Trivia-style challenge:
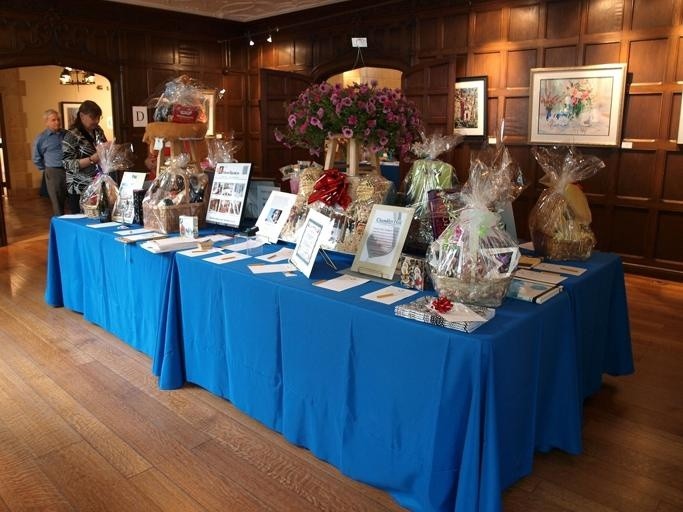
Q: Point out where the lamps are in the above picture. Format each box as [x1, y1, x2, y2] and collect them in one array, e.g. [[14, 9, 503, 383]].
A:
[[59, 66, 95, 93]]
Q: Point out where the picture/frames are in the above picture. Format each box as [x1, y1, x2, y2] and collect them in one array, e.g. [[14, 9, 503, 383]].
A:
[[62, 102, 83, 131], [453, 76, 488, 140], [195, 90, 217, 139], [527, 63, 627, 150]]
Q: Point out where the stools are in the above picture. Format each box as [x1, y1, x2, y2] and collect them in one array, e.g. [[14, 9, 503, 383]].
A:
[[146, 122, 208, 177], [323, 133, 381, 177]]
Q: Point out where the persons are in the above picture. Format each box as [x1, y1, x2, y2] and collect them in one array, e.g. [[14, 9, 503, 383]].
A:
[[270, 209, 281, 222], [400, 262, 421, 289], [32, 109, 78, 215], [61, 100, 108, 213]]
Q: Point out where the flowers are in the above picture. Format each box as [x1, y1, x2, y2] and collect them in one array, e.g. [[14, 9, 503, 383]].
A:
[[273, 79, 425, 161]]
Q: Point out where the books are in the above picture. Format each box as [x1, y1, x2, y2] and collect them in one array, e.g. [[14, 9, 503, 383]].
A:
[[140, 237, 198, 254], [394, 296, 495, 334], [114, 233, 162, 243], [505, 276, 563, 304], [517, 256, 540, 269]]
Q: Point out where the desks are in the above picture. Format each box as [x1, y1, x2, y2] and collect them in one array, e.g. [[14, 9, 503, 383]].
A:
[[44, 212, 635, 512]]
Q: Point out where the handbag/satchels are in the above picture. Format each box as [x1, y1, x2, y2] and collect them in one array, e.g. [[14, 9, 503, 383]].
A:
[[39, 172, 49, 198]]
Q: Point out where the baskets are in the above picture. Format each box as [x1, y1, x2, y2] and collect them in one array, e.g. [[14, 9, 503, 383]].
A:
[[404, 193, 495, 250], [431, 269, 512, 307], [79, 194, 114, 218], [530, 225, 594, 260], [141, 174, 209, 233]]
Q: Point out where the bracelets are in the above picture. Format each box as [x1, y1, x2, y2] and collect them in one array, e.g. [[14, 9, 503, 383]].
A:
[[89, 157, 95, 165]]
